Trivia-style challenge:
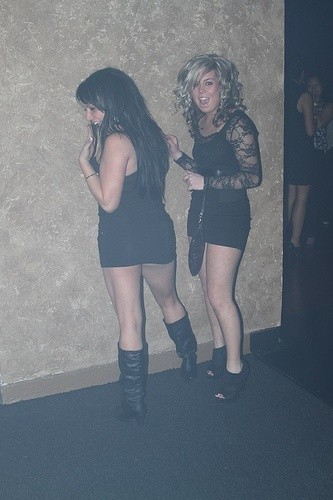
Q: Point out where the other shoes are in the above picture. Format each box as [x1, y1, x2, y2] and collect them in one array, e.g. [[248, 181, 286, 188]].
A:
[[284, 241, 316, 257]]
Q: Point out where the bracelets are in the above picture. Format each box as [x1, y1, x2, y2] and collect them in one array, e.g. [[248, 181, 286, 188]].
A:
[[85, 172, 98, 180]]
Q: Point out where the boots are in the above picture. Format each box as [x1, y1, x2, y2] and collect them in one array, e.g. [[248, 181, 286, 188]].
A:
[[163, 312, 196, 385], [106, 342, 148, 426]]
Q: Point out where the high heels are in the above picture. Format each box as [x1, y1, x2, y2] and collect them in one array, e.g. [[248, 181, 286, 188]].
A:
[[214, 360, 249, 401], [204, 344, 227, 378]]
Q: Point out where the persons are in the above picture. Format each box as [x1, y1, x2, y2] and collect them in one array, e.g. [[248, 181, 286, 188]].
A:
[[285, 55, 333, 260], [162, 55, 261, 404], [74, 68, 197, 422]]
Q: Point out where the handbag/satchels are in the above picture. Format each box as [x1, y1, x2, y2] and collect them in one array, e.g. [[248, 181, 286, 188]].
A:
[[187, 224, 205, 273]]
